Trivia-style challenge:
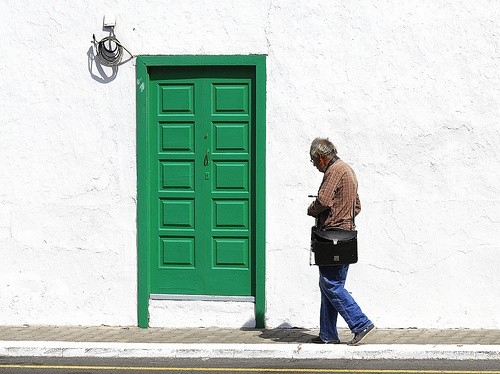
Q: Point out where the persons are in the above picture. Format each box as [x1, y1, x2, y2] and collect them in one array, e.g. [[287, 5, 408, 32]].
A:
[[308, 137, 375, 345]]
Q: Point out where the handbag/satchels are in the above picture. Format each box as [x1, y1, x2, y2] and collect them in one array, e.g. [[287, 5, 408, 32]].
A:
[[313, 230, 357, 266]]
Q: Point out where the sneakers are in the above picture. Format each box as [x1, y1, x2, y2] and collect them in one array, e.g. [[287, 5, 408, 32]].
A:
[[312, 336, 340, 344], [348, 324, 375, 346]]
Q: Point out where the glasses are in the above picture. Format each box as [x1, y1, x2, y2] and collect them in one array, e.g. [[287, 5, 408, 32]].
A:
[[310, 157, 319, 164]]
